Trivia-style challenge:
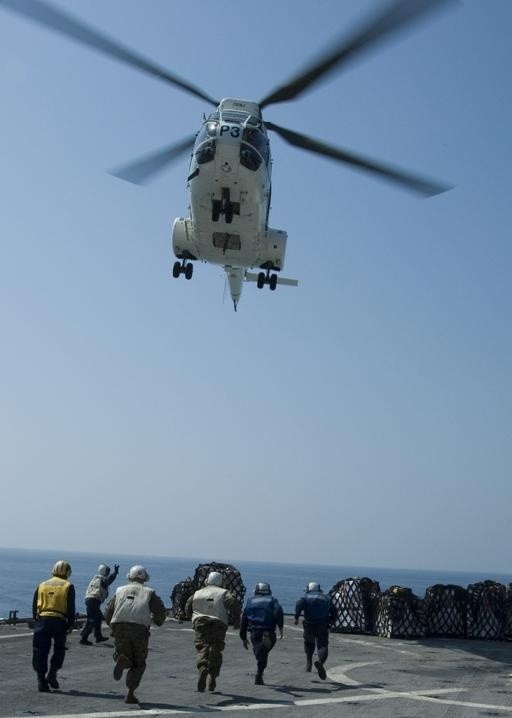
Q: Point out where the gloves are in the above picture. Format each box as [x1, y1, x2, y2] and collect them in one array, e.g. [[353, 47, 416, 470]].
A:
[[114, 563, 120, 573]]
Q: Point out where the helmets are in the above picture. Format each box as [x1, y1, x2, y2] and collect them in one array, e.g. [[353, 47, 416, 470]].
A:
[[205, 571, 225, 586], [51, 561, 72, 578], [98, 565, 110, 576], [307, 582, 321, 592], [255, 583, 272, 595], [127, 565, 149, 583]]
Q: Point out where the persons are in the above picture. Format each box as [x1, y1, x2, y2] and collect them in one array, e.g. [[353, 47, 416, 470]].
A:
[[239, 581, 286, 685], [295, 582, 339, 681], [31, 560, 77, 693], [79, 563, 121, 646], [102, 564, 166, 704], [184, 570, 241, 692]]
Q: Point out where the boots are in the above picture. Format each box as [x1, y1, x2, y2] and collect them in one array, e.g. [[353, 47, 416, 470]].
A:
[[197, 665, 208, 691], [209, 677, 215, 691], [124, 686, 140, 704], [314, 661, 326, 680], [79, 636, 93, 646], [95, 635, 109, 643], [305, 660, 312, 672], [113, 655, 126, 680], [47, 666, 60, 689], [37, 671, 50, 691], [254, 670, 264, 685]]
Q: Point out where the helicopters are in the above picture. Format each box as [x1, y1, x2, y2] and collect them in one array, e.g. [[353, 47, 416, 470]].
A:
[[0, 0, 467, 314]]
